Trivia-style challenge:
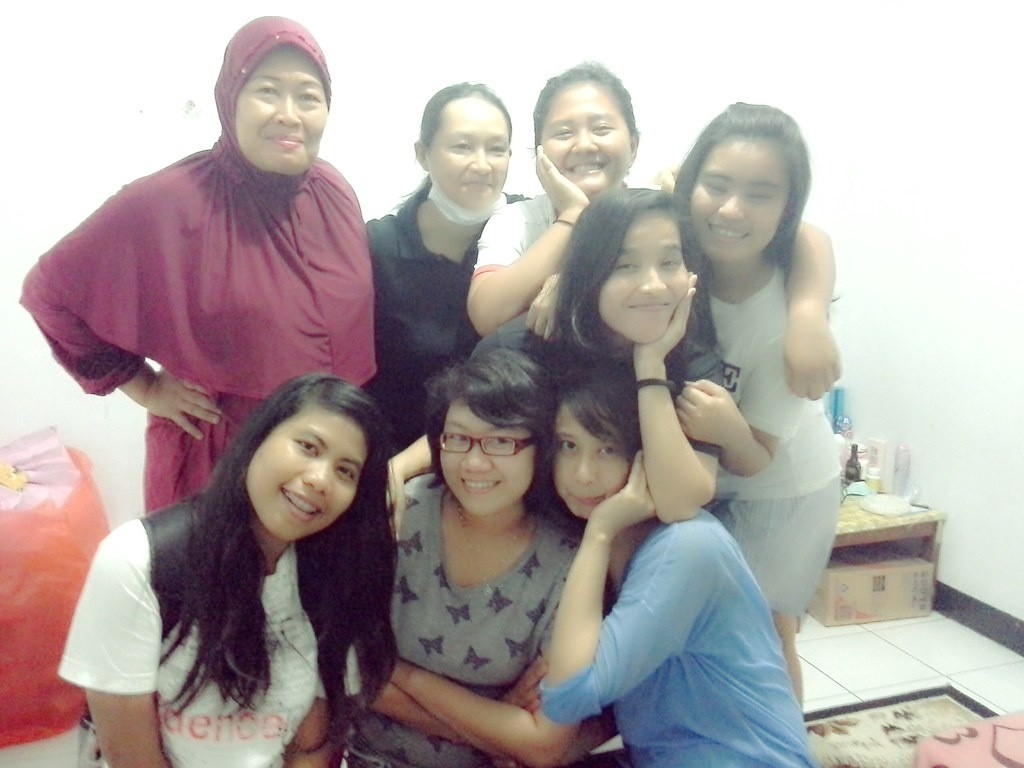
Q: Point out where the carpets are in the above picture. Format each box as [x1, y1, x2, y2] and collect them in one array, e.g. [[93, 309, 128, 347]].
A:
[[591, 686, 1000, 766]]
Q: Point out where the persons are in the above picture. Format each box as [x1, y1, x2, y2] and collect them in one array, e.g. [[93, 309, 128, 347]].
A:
[[382, 184, 723, 542], [523, 102, 846, 717], [535, 346, 815, 768], [345, 346, 579, 768], [306, 78, 686, 714], [59, 372, 403, 768], [17, 13, 380, 518], [466, 62, 846, 401]]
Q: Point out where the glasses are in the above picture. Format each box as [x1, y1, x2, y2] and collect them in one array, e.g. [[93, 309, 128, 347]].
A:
[[439, 433, 535, 456]]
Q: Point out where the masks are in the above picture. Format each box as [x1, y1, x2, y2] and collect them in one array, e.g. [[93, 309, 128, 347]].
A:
[[427, 151, 507, 225]]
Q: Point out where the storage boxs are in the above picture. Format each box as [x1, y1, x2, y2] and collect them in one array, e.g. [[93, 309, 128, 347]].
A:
[[805, 540, 936, 628]]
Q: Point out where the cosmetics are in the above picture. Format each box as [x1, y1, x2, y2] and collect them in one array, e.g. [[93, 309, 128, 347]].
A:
[[843, 437, 910, 499]]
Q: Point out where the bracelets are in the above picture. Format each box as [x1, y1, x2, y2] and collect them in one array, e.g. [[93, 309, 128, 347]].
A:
[[551, 220, 573, 227], [635, 378, 674, 391]]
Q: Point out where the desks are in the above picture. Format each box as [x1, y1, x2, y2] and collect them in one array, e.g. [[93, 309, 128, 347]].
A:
[[834, 499, 946, 564]]
